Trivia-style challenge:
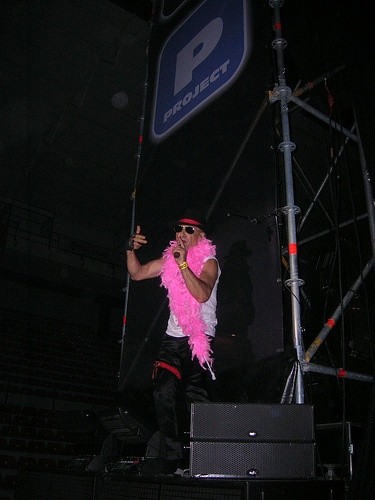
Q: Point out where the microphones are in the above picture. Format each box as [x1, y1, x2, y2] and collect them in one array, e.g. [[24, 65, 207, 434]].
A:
[[174, 239, 185, 257]]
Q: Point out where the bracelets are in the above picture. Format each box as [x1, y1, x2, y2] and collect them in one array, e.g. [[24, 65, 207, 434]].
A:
[[179, 261, 189, 271]]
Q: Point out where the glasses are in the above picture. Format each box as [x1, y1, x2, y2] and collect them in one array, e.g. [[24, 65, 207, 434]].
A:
[[173, 225, 198, 234]]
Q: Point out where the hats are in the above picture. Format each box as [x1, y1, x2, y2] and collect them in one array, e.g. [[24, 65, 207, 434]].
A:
[[170, 209, 210, 234]]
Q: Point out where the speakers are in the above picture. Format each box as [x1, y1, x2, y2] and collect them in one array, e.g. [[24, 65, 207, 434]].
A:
[[178, 404, 319, 477]]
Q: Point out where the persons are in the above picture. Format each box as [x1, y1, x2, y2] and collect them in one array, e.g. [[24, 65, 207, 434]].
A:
[[122, 214, 222, 474]]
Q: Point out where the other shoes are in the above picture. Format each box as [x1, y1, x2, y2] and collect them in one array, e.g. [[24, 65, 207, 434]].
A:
[[126, 458, 156, 473]]
